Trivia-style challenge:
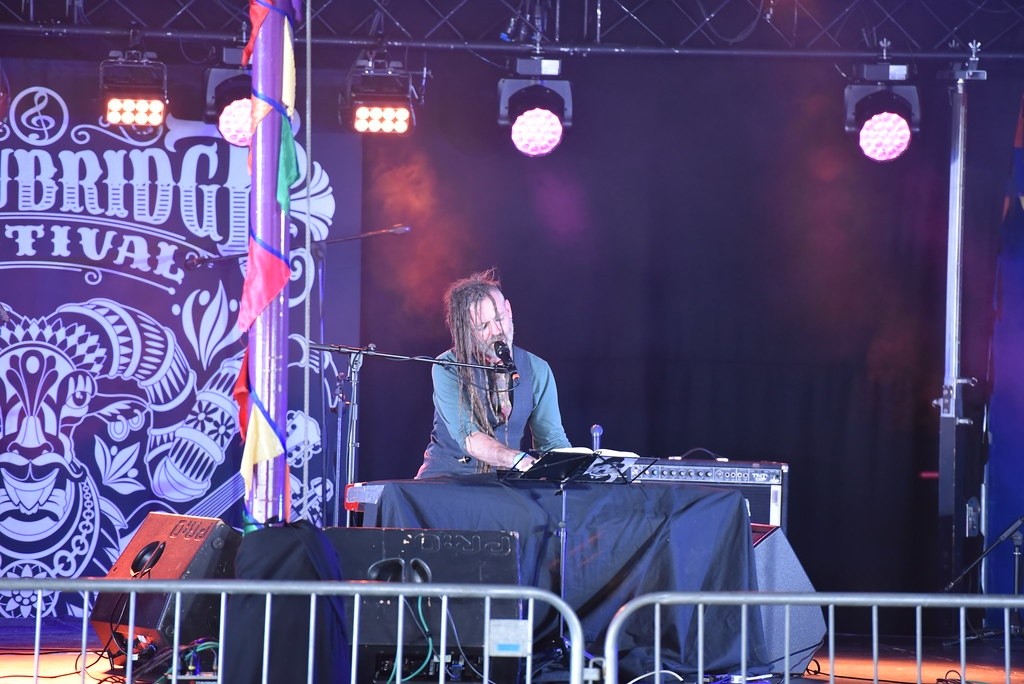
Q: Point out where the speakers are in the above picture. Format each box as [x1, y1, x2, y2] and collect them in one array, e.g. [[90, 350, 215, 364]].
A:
[[751, 524, 827, 675], [88, 510, 243, 677], [323, 526, 524, 684], [627, 456, 788, 532]]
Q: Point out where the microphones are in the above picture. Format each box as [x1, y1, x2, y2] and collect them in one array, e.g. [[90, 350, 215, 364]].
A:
[[184, 257, 204, 270], [494, 341, 521, 384]]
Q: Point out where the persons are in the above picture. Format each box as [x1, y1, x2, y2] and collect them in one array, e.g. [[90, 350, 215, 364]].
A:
[[413, 275, 572, 480]]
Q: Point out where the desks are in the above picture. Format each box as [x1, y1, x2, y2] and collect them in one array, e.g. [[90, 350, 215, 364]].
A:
[[374, 480, 776, 684]]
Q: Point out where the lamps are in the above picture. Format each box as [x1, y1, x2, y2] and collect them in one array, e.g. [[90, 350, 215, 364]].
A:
[[493, 54, 573, 159], [201, 44, 251, 147], [841, 57, 922, 162], [336, 48, 419, 137], [97, 47, 170, 127]]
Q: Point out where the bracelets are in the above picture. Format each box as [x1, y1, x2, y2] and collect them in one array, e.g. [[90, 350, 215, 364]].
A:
[[513, 453, 528, 468]]
